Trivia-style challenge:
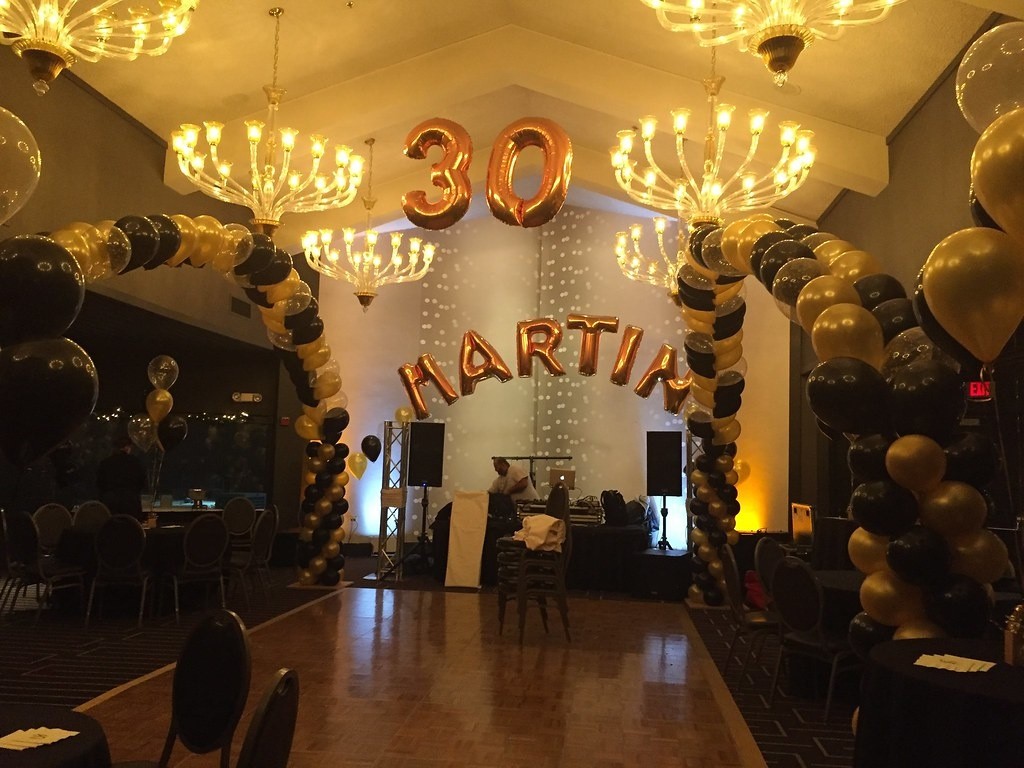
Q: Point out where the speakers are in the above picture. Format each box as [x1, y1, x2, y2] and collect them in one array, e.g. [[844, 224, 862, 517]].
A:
[[407, 422, 446, 488], [646, 431, 682, 497]]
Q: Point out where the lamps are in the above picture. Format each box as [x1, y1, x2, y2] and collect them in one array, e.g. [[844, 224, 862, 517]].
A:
[[0, 0, 196, 97], [640, 0, 905, 88], [614, 216, 691, 306], [170, 8, 365, 238], [301, 139, 436, 315], [609, 6, 818, 228]]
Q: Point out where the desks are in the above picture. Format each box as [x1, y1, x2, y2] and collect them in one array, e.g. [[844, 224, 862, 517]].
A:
[[633, 549, 690, 599], [812, 569, 865, 631], [0, 699, 111, 768], [140, 507, 265, 527]]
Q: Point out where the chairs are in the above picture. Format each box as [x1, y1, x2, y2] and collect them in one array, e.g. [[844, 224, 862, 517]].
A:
[[111, 608, 250, 768], [0, 496, 279, 625], [236, 667, 298, 768], [720, 537, 865, 727], [496, 482, 573, 644]]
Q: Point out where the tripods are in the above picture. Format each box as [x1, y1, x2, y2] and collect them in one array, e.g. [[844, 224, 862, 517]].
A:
[[654, 496, 674, 549], [380, 486, 432, 580]]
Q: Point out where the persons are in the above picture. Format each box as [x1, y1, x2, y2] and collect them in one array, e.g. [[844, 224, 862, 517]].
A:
[[97, 439, 148, 520], [488, 457, 539, 501]]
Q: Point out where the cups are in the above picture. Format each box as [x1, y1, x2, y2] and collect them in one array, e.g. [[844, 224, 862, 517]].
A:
[[160, 494, 172, 509], [149, 519, 156, 528]]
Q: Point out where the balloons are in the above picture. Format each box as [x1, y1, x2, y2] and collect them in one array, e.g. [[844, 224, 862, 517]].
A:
[[957, 21, 1024, 135], [402, 116, 572, 232], [362, 435, 381, 463], [348, 453, 367, 480], [677, 214, 1009, 738], [0, 104, 366, 586], [396, 407, 413, 428], [970, 184, 1005, 232], [922, 226, 1024, 365], [397, 314, 692, 421], [970, 107, 1023, 250]]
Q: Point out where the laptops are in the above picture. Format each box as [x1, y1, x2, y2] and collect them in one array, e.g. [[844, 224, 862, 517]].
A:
[[549, 468, 576, 490]]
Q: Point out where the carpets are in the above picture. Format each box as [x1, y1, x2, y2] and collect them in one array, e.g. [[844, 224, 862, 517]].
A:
[[342, 557, 444, 590], [686, 606, 857, 768], [0, 585, 350, 717]]
